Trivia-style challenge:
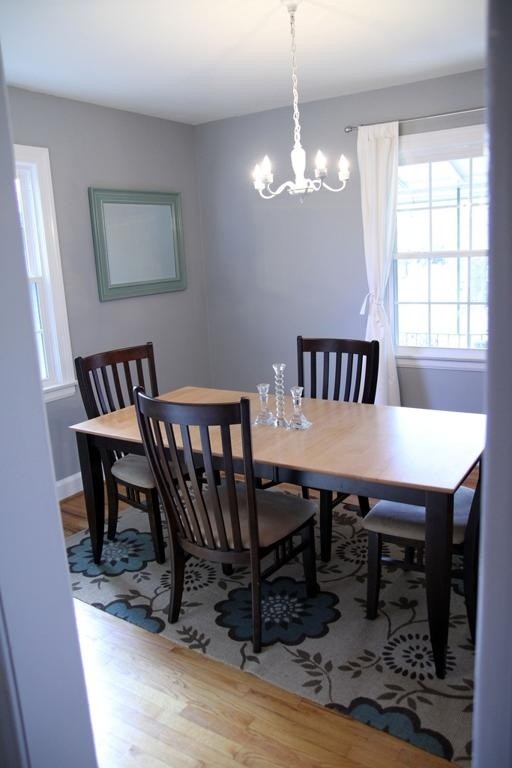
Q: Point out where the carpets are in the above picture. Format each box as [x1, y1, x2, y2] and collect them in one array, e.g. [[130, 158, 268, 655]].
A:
[[66, 472, 475, 766]]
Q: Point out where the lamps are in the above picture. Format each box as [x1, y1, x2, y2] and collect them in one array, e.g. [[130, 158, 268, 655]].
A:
[[251, 1, 350, 202]]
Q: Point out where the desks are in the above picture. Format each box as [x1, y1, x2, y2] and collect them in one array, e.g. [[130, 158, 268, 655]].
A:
[[68, 386, 487, 680]]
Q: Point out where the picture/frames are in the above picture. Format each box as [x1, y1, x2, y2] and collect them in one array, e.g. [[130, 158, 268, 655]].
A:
[[87, 187, 188, 303]]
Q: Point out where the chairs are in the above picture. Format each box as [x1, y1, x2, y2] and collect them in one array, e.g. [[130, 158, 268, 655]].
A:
[[130, 386, 320, 655], [297, 336, 379, 554], [75, 342, 221, 564], [363, 459, 481, 648]]
